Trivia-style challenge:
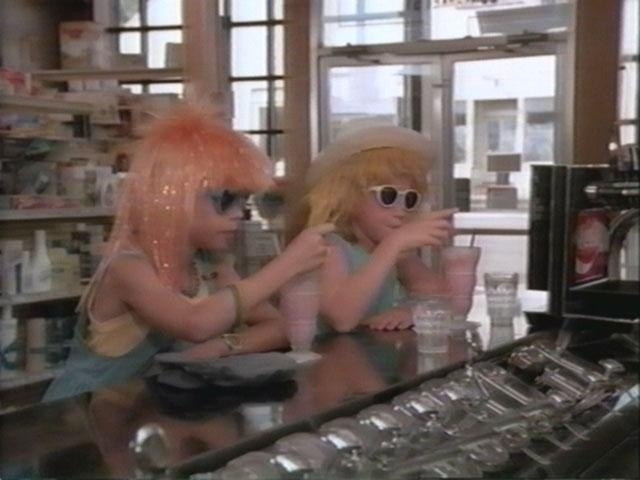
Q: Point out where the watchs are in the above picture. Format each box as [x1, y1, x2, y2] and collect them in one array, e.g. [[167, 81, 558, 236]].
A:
[[221, 333, 242, 353]]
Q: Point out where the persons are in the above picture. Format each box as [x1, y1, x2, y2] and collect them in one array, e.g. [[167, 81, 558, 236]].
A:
[[284, 324, 469, 425], [41, 112, 337, 405], [82, 383, 243, 479], [279, 124, 460, 335]]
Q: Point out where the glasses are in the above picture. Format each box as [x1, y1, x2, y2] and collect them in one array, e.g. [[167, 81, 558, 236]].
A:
[[361, 184, 422, 210], [205, 190, 235, 211]]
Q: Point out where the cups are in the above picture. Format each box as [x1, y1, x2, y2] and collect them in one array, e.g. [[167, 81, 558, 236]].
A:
[[280, 278, 322, 354], [409, 246, 520, 354]]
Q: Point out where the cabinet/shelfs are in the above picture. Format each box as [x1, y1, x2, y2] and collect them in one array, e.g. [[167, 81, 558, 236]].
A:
[[0, 88, 148, 418]]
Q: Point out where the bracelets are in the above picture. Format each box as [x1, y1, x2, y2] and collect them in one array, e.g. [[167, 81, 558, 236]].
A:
[[227, 280, 243, 331]]
